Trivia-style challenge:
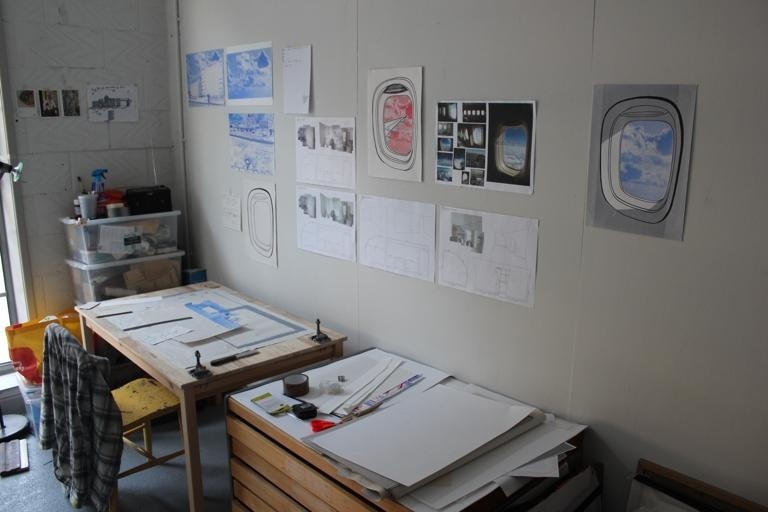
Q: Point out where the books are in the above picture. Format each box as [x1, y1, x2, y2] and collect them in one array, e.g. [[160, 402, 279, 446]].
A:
[[16, 371, 43, 440], [61, 211, 187, 309]]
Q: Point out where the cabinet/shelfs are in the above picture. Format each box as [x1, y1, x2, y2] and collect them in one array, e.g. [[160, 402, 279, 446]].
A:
[[73, 199, 81, 219], [106, 203, 124, 217], [87, 248, 97, 265]]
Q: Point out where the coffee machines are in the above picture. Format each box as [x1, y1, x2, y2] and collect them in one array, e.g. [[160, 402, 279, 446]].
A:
[[312, 420, 340, 432]]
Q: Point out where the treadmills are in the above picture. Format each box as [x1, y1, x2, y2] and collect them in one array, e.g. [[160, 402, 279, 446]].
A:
[[292, 402, 317, 421]]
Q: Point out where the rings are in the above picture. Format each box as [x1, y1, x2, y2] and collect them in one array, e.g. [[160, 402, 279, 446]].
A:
[[339, 400, 383, 424], [122, 316, 192, 332], [78, 176, 96, 194], [96, 311, 132, 318]]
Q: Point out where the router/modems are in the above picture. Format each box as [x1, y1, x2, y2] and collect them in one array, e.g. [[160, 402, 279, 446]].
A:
[[283, 373, 309, 398]]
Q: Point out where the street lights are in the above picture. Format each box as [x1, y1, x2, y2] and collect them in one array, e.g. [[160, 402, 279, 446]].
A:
[[5, 311, 83, 385]]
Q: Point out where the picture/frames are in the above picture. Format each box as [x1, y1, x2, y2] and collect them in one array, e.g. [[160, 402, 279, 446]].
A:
[[74, 281, 348, 512], [224, 347, 602, 511]]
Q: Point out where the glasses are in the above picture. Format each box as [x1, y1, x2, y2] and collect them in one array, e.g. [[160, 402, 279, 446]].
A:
[[1, 159, 29, 440]]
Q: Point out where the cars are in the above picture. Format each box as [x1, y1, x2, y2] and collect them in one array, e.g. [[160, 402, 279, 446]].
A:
[[77, 194, 100, 221]]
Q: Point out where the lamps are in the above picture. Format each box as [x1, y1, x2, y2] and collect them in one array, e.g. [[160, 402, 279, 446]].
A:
[[44, 324, 184, 512]]
